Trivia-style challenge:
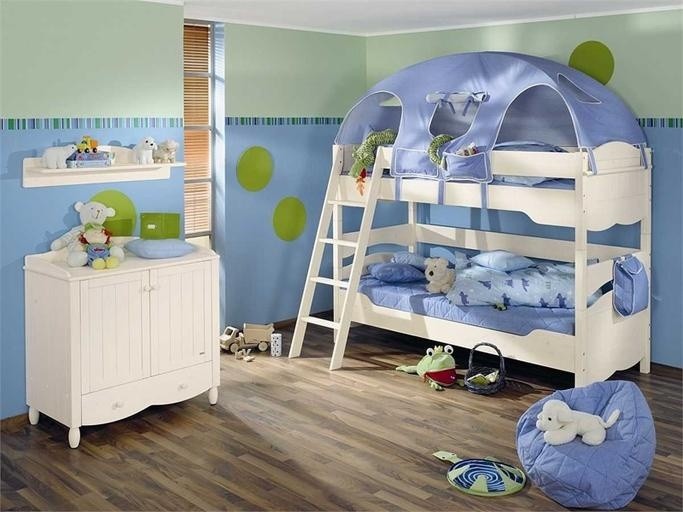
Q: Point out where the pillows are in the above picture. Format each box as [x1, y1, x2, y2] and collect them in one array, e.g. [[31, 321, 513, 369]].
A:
[[469, 248, 536, 273], [122, 238, 196, 260], [366, 250, 432, 284]]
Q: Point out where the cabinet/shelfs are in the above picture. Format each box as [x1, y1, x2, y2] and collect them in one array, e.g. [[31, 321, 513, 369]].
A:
[[20, 235, 221, 449]]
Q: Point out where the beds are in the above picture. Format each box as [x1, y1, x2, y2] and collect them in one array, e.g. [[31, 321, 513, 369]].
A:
[[326, 142, 653, 393]]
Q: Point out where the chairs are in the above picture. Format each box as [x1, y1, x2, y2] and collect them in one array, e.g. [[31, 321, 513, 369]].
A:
[[516, 379, 655, 510]]
